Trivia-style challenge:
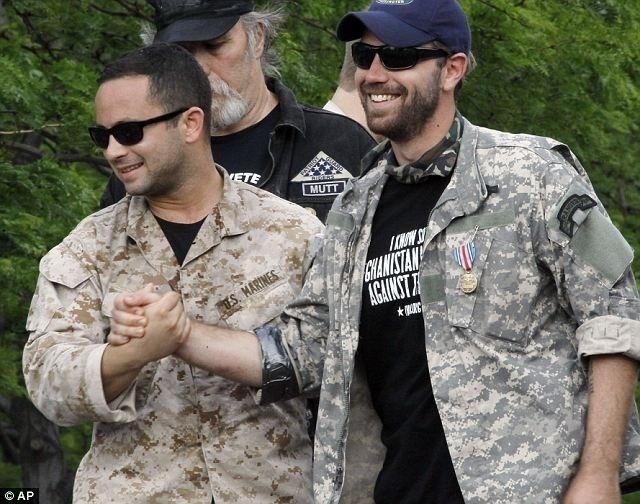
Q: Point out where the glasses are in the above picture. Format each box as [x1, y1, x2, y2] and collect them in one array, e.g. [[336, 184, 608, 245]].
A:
[[351, 42, 449, 72], [88, 107, 188, 150]]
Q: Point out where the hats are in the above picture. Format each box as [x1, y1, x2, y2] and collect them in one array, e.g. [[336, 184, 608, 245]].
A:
[[154, 0, 253, 43], [337, 1, 472, 55]]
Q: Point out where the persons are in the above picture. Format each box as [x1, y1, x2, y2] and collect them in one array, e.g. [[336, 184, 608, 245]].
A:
[[20, 41, 326, 504], [103, 0, 640, 503], [322, 3, 391, 146], [98, 0, 381, 224]]
